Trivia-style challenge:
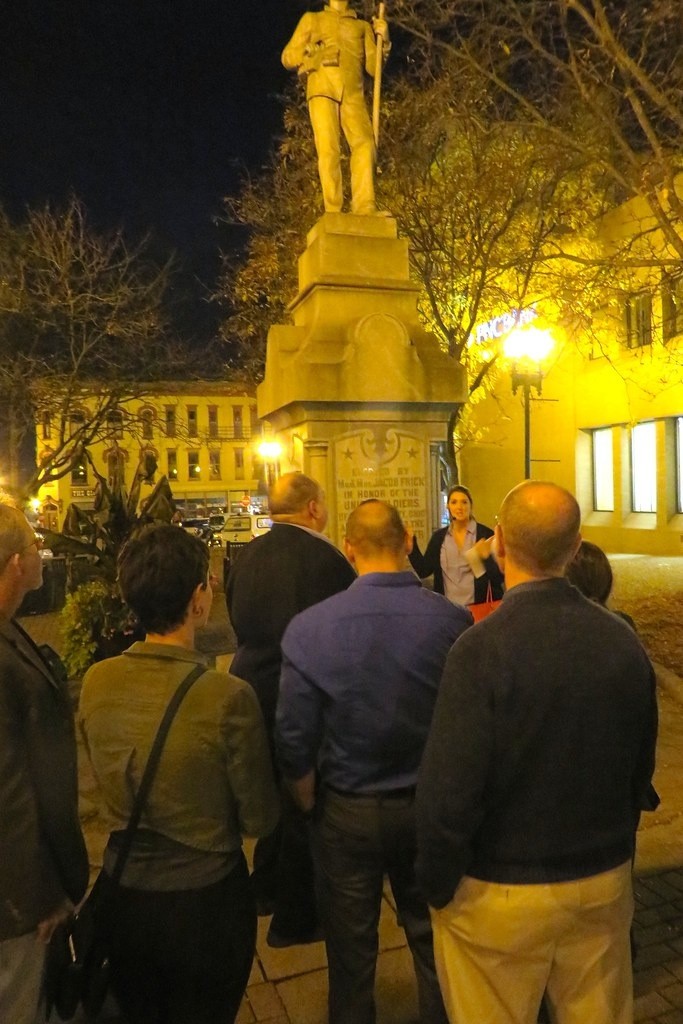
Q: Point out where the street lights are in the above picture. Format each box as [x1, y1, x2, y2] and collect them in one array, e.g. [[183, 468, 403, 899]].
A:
[[508, 329, 556, 477]]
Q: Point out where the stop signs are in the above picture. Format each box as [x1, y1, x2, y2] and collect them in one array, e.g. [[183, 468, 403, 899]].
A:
[[242, 496, 250, 506]]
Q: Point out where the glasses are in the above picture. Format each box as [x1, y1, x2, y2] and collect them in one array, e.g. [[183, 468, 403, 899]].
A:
[[4, 532, 45, 561]]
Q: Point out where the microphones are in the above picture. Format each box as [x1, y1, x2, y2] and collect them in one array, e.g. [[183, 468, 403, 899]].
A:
[[448, 508, 457, 521]]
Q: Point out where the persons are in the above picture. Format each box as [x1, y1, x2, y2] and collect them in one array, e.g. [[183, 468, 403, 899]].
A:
[[405, 484, 506, 608], [70, 516, 286, 1024], [269, 496, 477, 1024], [0, 492, 94, 1024], [280, 0, 394, 221], [223, 471, 360, 948], [406, 479, 657, 1024], [553, 538, 661, 964], [170, 509, 184, 527]]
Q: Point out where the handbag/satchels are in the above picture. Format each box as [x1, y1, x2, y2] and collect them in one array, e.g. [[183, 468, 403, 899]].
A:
[[46, 884, 113, 1018]]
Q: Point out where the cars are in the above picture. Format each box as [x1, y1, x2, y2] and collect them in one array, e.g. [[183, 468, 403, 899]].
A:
[[170, 512, 273, 547]]
